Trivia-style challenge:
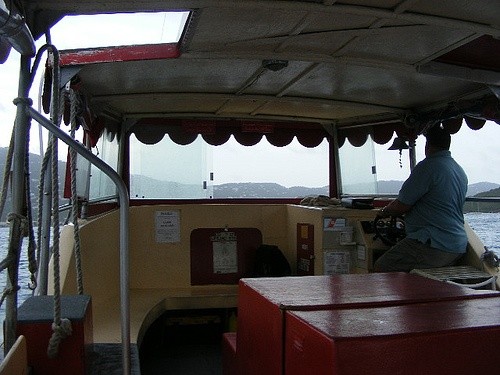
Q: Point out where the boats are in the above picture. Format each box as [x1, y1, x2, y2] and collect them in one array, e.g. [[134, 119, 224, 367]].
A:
[[0, 0, 499, 375]]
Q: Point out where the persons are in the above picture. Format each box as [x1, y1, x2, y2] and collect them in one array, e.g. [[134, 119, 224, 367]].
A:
[[369, 126, 468, 273]]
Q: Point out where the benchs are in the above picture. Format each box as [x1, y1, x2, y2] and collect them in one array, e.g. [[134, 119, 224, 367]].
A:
[[2, 293, 93, 374]]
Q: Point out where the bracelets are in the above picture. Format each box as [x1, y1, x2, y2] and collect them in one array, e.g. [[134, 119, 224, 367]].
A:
[[381, 206, 391, 217]]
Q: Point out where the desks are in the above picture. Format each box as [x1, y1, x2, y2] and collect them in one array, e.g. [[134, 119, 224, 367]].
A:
[[222, 264, 500, 375]]
